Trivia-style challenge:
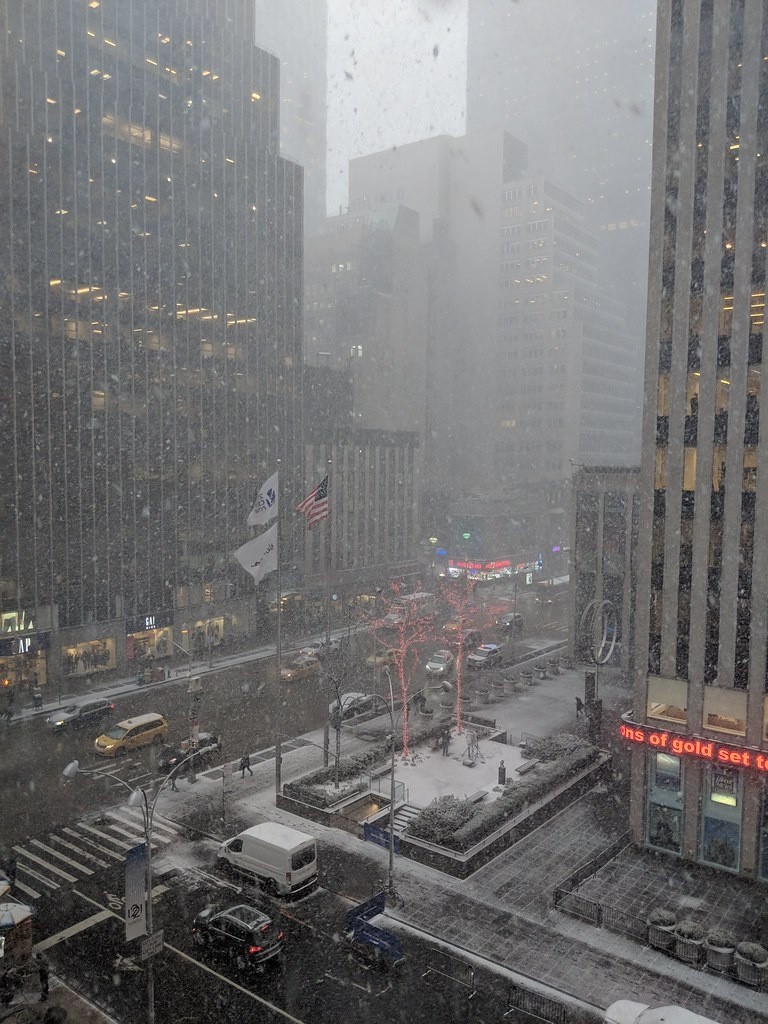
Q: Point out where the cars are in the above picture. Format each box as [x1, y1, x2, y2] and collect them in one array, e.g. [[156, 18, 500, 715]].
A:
[[280, 656, 322, 683], [365, 648, 403, 671], [300, 639, 342, 661], [329, 692, 378, 719], [154, 732, 221, 774], [426, 650, 454, 679]]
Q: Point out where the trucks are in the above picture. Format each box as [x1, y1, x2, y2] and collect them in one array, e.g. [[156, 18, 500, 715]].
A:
[[384, 592, 437, 632]]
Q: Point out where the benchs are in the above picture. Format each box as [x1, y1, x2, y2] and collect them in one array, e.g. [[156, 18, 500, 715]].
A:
[[515, 758, 540, 776], [369, 763, 397, 779], [428, 737, 454, 751], [463, 789, 490, 805]]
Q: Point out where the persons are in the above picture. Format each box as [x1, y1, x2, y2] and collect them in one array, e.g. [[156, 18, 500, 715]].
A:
[[1, 619, 220, 713], [168, 766, 178, 792], [239, 749, 253, 778], [404, 687, 426, 722], [442, 727, 450, 756], [574, 696, 586, 718], [34, 952, 52, 1001]]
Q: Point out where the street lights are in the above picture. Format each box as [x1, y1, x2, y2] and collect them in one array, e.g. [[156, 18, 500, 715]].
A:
[[353, 663, 453, 890], [62, 743, 218, 934], [160, 612, 233, 679]]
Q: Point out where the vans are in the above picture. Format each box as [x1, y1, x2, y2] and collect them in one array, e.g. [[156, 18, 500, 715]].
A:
[[495, 613, 524, 631], [92, 711, 168, 758], [215, 821, 320, 897]]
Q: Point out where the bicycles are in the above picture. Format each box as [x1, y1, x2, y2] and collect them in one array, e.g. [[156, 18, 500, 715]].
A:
[[370, 878, 405, 907]]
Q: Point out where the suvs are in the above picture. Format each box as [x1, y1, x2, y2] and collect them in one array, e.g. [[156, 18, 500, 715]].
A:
[[46, 697, 116, 733], [467, 643, 504, 670], [189, 904, 288, 970]]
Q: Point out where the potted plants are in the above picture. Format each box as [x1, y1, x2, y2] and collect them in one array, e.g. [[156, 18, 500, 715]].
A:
[[419, 653, 572, 724], [644, 907, 768, 987]]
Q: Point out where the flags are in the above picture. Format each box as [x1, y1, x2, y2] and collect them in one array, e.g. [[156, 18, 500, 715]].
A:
[[246, 471, 279, 526], [233, 523, 279, 586], [296, 476, 330, 528]]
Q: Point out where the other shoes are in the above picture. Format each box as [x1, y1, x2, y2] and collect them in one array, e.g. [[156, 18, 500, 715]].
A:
[[241, 776, 244, 778], [251, 771, 253, 776], [38, 996, 48, 1001]]
